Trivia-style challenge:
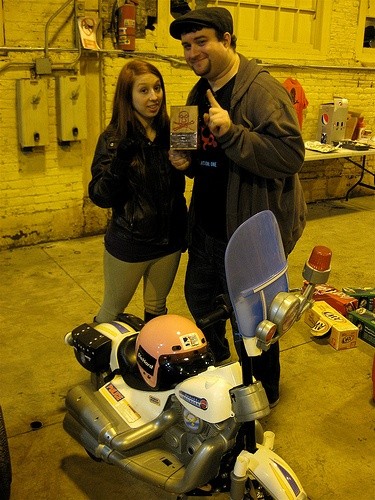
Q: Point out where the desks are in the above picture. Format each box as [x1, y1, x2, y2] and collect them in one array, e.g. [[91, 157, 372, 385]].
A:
[[304, 146, 375, 202]]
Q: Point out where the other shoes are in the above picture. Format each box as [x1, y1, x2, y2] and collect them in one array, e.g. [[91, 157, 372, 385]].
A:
[[208, 339, 231, 365], [267, 393, 279, 408]]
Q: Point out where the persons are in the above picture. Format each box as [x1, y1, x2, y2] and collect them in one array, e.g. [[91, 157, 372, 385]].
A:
[[88, 59, 189, 325], [169, 6, 306, 409]]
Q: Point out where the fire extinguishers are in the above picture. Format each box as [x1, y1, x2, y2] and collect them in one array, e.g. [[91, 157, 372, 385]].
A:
[[117, 4, 136, 50]]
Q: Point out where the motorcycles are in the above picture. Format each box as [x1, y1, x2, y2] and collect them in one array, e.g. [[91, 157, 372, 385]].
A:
[[61, 211, 333, 500]]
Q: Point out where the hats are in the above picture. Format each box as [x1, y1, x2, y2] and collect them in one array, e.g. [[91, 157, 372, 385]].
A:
[[169, 7, 233, 40]]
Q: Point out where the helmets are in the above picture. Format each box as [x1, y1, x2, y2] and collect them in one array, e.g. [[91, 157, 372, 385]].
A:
[[135, 315, 207, 388]]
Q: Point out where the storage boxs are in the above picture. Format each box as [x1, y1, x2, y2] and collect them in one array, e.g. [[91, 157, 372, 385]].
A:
[[341, 287, 375, 313], [304, 301, 358, 351], [318, 96, 348, 142], [303, 280, 358, 316], [348, 308, 375, 347]]
